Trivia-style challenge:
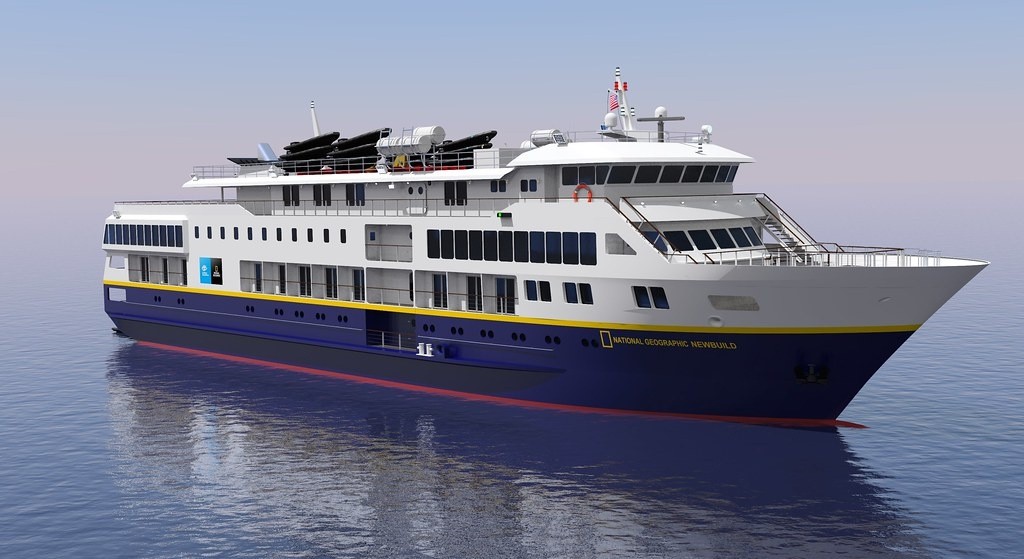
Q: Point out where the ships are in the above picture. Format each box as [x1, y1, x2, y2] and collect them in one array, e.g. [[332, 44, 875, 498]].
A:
[[103, 59, 993, 432]]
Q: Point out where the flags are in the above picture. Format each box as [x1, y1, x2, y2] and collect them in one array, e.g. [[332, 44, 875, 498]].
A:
[[610, 94, 619, 111]]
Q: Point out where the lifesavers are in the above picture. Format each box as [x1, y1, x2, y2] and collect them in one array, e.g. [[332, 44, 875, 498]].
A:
[[574, 184, 592, 202]]
